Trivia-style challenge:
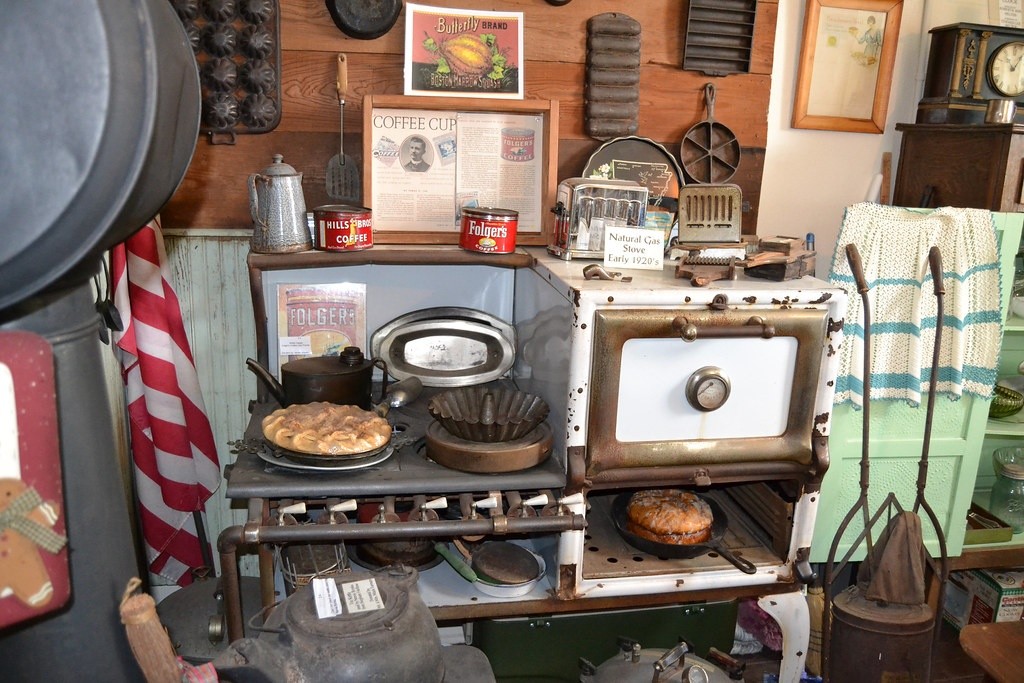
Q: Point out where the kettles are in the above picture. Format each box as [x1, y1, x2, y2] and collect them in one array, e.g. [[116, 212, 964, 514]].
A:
[[247, 346, 389, 410], [210, 560, 447, 683]]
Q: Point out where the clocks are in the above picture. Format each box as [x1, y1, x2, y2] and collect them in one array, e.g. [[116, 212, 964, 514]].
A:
[[987, 40, 1024, 97]]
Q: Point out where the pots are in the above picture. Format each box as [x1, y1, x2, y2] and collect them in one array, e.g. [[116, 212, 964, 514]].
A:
[[263, 377, 424, 467], [612, 489, 757, 574], [356, 501, 477, 583], [681, 84, 740, 184]]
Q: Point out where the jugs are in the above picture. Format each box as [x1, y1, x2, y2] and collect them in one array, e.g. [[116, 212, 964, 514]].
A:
[[249, 152, 314, 254]]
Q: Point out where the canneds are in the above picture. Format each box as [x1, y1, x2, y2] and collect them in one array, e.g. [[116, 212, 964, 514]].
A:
[[314, 203, 374, 253], [499, 128, 536, 162], [458, 207, 518, 255]]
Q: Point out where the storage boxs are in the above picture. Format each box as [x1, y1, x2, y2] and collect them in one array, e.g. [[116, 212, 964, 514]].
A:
[[940, 567, 1024, 638]]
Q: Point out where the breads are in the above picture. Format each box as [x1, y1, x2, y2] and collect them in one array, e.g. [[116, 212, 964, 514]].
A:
[[628, 490, 714, 550], [262, 401, 392, 456]]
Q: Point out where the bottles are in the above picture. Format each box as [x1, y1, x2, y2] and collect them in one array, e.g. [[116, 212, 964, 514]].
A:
[[989, 446, 1024, 534]]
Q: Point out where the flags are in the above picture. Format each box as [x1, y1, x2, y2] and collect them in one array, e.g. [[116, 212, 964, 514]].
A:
[[107, 203, 222, 592]]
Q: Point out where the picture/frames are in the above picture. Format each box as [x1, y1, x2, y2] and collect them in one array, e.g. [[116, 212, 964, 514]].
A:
[[403, 1, 525, 100], [791, 0, 906, 134], [360, 93, 559, 243]]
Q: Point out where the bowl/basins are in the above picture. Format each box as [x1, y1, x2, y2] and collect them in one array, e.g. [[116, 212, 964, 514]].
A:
[[466, 550, 547, 597], [988, 384, 1024, 418]]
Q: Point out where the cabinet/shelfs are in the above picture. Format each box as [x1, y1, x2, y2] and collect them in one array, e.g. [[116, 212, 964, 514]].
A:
[[512, 243, 849, 605], [895, 119, 1024, 221], [806, 206, 1023, 583]]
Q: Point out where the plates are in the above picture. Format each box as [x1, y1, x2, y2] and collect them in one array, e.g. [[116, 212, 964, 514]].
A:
[[258, 445, 395, 471]]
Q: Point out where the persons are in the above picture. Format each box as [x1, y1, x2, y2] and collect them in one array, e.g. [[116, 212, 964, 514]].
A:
[[402, 136, 432, 171], [855, 15, 883, 63]]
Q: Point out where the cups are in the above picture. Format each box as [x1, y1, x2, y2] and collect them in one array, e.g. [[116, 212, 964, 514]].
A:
[[983, 98, 1018, 123]]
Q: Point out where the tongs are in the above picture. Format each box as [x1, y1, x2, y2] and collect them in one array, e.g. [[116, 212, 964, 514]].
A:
[[968, 509, 1003, 531]]
